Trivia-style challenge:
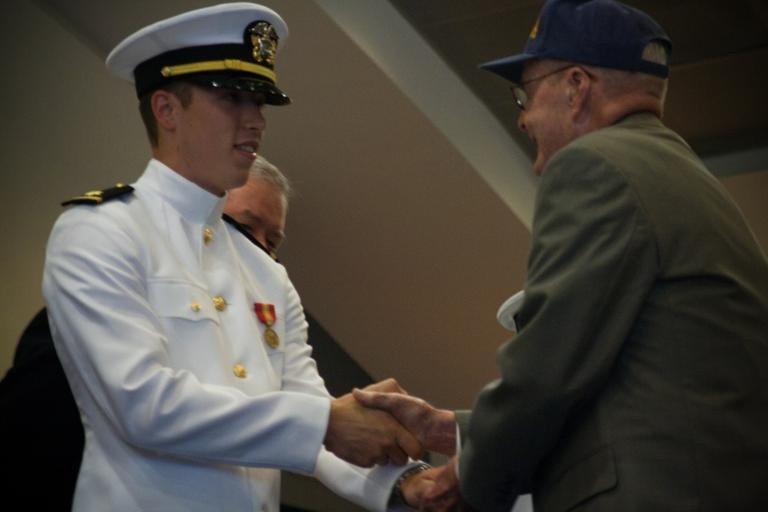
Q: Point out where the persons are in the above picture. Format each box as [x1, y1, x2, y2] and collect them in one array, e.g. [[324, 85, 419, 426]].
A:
[[494, 288, 534, 512], [0, 153, 431, 511], [38, 2, 450, 512], [349, 2, 768, 512]]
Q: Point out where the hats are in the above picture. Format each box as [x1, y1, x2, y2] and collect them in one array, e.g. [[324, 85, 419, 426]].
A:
[[104, 2, 292, 107], [478, 1, 672, 85]]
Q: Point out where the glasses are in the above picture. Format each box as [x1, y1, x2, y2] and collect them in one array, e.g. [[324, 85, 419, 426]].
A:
[[509, 61, 599, 111]]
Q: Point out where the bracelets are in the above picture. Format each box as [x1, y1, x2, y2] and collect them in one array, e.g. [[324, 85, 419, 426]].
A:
[[393, 464, 431, 495]]
